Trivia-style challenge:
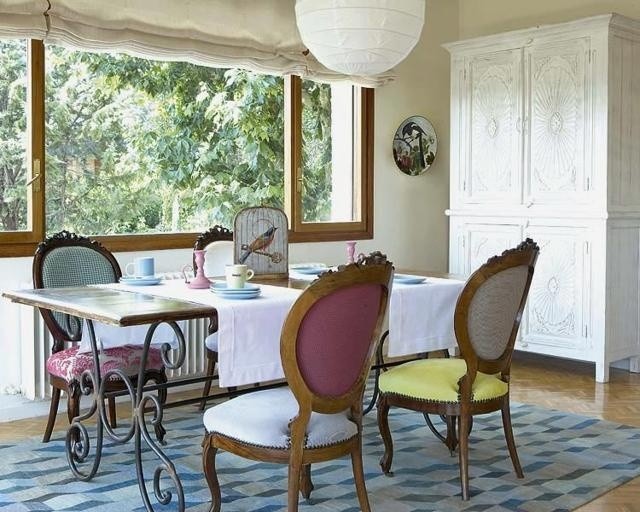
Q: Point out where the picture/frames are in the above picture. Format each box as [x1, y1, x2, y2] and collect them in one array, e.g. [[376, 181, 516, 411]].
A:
[[232, 206, 290, 281]]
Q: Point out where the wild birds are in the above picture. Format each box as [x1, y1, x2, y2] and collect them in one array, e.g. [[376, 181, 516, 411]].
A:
[[238, 226, 278, 265], [402, 120, 427, 169]]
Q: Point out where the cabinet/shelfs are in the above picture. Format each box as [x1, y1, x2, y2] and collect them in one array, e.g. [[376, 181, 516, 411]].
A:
[[438, 11, 640, 384]]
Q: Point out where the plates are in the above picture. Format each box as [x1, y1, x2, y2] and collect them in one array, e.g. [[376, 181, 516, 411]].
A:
[[394, 275, 425, 284], [391, 113, 439, 176], [119, 276, 162, 285], [210, 284, 261, 301], [293, 266, 326, 274]]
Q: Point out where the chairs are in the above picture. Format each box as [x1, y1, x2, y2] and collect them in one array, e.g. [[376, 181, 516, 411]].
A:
[[32, 229, 168, 463], [192, 226, 265, 411], [200, 250, 395, 512], [375, 238, 541, 502]]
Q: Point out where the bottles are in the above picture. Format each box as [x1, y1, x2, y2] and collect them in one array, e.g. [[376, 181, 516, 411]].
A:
[[346, 240, 356, 266], [186, 250, 215, 289]]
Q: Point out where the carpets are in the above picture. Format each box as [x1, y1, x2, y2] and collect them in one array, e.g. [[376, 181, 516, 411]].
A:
[[0, 369, 640, 512]]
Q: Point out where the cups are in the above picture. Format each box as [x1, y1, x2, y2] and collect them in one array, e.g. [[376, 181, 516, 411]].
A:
[[126, 256, 154, 279], [226, 265, 256, 289]]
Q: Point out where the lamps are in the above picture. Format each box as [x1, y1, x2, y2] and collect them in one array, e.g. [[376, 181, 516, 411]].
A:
[[295, 0, 426, 80]]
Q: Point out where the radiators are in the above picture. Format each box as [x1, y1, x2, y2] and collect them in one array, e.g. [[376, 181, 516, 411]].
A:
[[5, 270, 214, 402]]
